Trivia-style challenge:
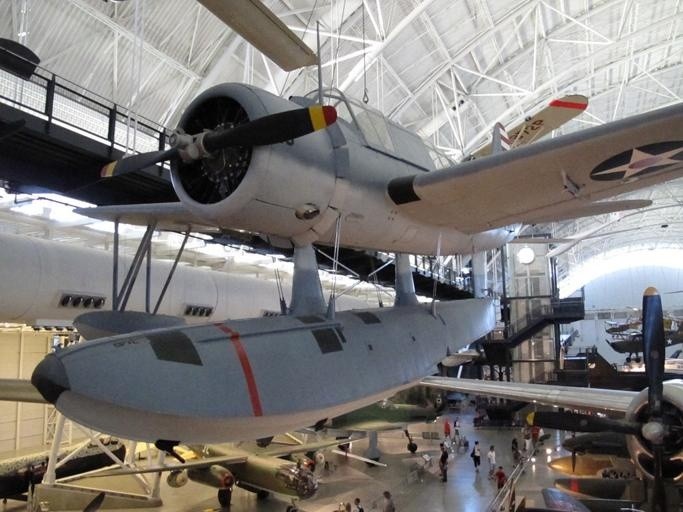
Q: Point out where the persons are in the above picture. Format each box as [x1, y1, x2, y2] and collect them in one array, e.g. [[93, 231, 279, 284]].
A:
[[438, 447, 447, 482], [473, 440, 480, 471], [538, 427, 544, 438], [531, 425, 538, 448], [523, 428, 530, 451], [335, 501, 352, 511], [352, 497, 363, 511], [381, 490, 395, 511], [520, 423, 527, 440], [494, 465, 506, 492], [471, 408, 481, 425], [437, 443, 444, 476], [443, 420, 450, 440], [453, 416, 459, 427], [487, 445, 495, 478], [510, 434, 520, 467]]
